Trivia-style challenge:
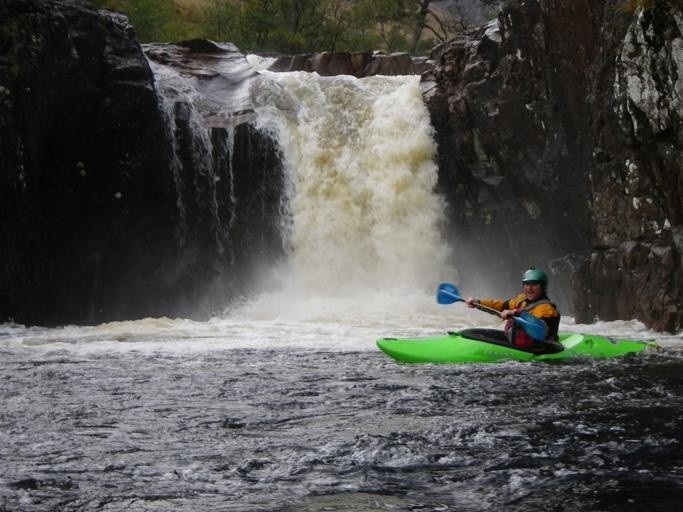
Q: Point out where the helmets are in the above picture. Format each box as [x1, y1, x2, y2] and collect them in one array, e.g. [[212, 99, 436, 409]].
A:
[[521, 266, 548, 286]]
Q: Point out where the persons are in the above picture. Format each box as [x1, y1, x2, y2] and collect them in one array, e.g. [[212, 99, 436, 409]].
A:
[[466, 268, 561, 351]]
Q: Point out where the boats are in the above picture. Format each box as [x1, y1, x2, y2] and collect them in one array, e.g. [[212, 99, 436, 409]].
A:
[[377, 326, 655, 365]]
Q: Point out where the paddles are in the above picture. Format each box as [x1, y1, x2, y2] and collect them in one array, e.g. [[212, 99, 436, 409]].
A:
[[437, 283, 549, 340]]
